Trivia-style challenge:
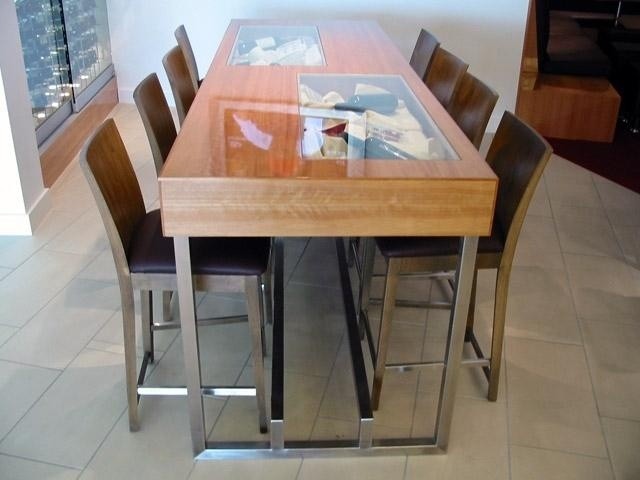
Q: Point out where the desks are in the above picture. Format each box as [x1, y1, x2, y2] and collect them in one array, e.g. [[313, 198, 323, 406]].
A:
[[154, 18, 500, 464]]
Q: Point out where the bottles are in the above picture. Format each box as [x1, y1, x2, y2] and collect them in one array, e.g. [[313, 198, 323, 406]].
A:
[[237, 35, 299, 56], [335, 93, 398, 113], [335, 129, 421, 161]]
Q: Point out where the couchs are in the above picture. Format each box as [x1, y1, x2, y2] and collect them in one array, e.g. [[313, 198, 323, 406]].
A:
[[515, 0, 622, 147]]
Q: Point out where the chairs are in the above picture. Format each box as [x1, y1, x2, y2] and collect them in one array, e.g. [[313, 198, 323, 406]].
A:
[[76, 116, 270, 436], [357, 110, 553, 412]]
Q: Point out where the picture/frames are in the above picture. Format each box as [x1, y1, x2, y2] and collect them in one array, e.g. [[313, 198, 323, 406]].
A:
[[206, 95, 363, 180]]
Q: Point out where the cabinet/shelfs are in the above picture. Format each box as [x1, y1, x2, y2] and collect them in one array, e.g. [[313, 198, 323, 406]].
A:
[[16, 1, 121, 189]]
